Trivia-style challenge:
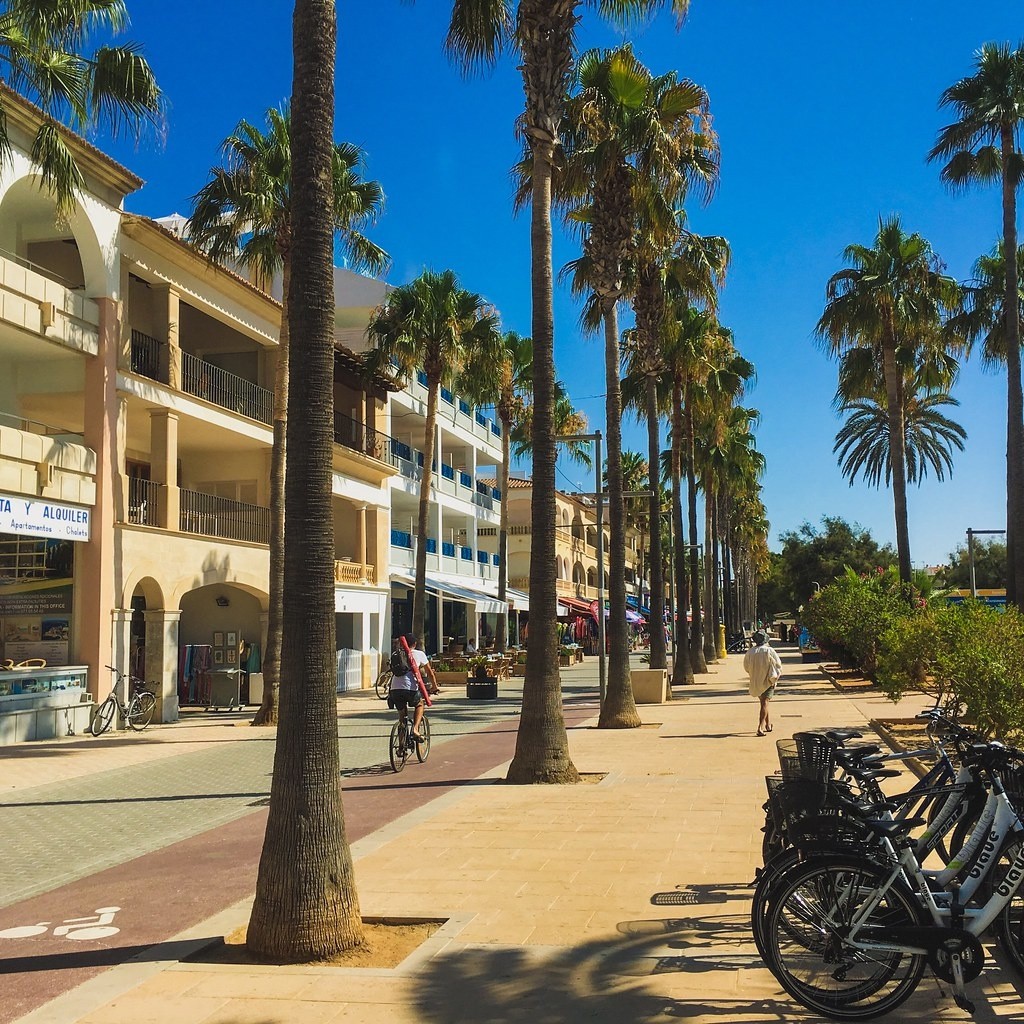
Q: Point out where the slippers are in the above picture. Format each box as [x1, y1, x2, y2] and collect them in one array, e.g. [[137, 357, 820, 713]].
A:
[[765, 723, 773, 732], [757, 733, 766, 736]]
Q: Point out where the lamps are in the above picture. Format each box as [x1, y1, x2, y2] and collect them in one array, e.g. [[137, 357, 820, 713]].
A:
[[216, 598, 229, 607]]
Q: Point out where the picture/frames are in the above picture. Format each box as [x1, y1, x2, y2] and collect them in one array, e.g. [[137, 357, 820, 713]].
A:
[[213, 649, 224, 664], [225, 649, 237, 664], [226, 631, 237, 647], [213, 632, 224, 647]]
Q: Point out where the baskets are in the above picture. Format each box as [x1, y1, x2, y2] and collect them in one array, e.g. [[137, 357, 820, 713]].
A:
[[765, 731, 841, 851]]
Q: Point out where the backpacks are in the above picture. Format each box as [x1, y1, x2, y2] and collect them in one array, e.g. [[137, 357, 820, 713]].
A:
[[389, 647, 411, 677]]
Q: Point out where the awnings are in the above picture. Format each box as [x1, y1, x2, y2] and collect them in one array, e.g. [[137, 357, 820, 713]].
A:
[[390, 572, 509, 614], [626, 610, 646, 624], [667, 611, 692, 621], [465, 587, 568, 616], [628, 602, 665, 619], [560, 597, 609, 616]]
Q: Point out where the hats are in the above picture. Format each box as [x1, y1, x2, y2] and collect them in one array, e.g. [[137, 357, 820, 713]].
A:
[[405, 632, 417, 643], [752, 629, 770, 645]]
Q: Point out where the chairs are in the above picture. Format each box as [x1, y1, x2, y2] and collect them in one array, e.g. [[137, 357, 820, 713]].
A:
[[129, 501, 147, 525], [434, 645, 583, 681]]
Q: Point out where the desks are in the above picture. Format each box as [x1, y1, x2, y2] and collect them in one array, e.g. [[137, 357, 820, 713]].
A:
[[494, 657, 512, 681], [181, 509, 217, 536]]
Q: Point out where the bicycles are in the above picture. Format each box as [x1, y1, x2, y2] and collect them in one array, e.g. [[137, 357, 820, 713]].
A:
[[747, 696, 1024, 1021], [375, 659, 393, 700], [389, 690, 437, 773], [91, 665, 157, 737]]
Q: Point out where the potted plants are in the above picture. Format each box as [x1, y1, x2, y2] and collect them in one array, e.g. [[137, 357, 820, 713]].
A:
[[466, 655, 497, 699], [434, 663, 473, 686], [512, 656, 526, 676], [561, 649, 575, 667]]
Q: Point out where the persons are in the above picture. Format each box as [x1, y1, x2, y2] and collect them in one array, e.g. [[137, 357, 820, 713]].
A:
[[637, 625, 644, 637], [390, 634, 438, 756], [780, 622, 788, 642], [467, 638, 478, 659], [744, 630, 781, 737], [663, 622, 670, 650]]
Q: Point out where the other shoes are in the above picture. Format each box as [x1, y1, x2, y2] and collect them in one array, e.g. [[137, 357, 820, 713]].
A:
[[397, 750, 408, 757], [410, 728, 425, 743]]
